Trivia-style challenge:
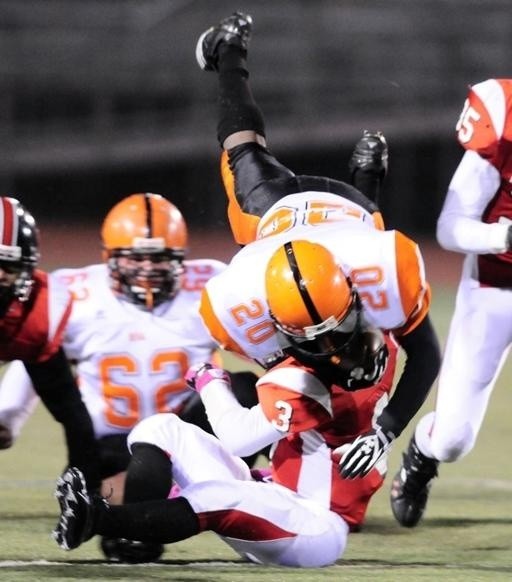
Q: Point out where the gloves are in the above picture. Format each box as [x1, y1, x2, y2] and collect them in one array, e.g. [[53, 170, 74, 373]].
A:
[[183, 362, 233, 392], [333, 421, 396, 480]]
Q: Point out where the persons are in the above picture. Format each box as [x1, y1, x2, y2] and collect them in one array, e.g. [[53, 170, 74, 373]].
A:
[[0, 191, 399, 566], [389, 80, 512, 525], [194, 13, 442, 479]]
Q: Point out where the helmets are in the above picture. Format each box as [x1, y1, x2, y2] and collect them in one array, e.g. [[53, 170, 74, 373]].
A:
[[265, 240, 389, 392], [0, 195, 39, 302], [102, 192, 187, 310]]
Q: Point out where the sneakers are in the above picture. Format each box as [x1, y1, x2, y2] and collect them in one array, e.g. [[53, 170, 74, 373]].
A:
[[102, 535, 164, 563], [390, 431, 440, 528], [196, 9, 252, 71], [51, 465, 109, 550], [349, 129, 389, 178]]
